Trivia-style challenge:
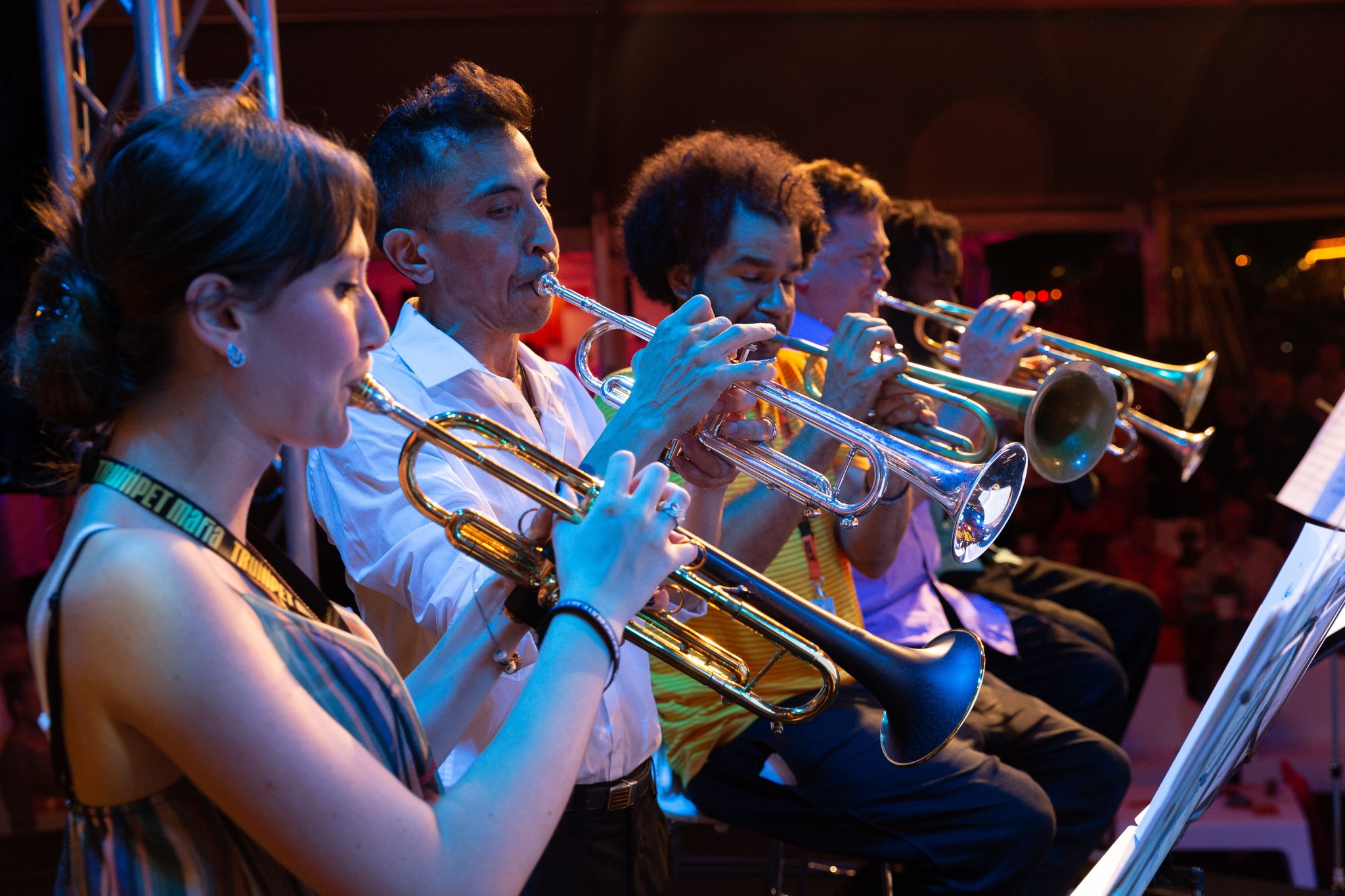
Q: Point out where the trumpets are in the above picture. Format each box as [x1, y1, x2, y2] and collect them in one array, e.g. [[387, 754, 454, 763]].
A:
[[531, 269, 1031, 563], [770, 328, 1119, 484], [871, 286, 1217, 430], [347, 371, 987, 771], [1107, 401, 1215, 485]]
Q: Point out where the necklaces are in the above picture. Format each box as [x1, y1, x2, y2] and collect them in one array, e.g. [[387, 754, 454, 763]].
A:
[[514, 356, 543, 420]]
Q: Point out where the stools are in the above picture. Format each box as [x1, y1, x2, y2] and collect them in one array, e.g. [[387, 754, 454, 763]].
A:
[[1119, 778, 1317, 887]]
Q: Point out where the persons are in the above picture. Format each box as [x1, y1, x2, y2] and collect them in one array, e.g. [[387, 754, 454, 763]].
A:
[[881, 196, 1162, 752], [0, 90, 697, 896], [796, 160, 1136, 750], [306, 61, 776, 896], [595, 134, 1132, 896]]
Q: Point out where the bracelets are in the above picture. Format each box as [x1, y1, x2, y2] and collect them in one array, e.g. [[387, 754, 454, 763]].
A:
[[863, 473, 911, 505], [537, 600, 620, 693], [473, 589, 522, 674]]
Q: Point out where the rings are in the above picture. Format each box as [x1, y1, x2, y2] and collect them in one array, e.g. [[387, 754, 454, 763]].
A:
[[659, 500, 686, 526]]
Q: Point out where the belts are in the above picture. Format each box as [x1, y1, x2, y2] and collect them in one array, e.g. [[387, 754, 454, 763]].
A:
[[566, 762, 657, 812]]
